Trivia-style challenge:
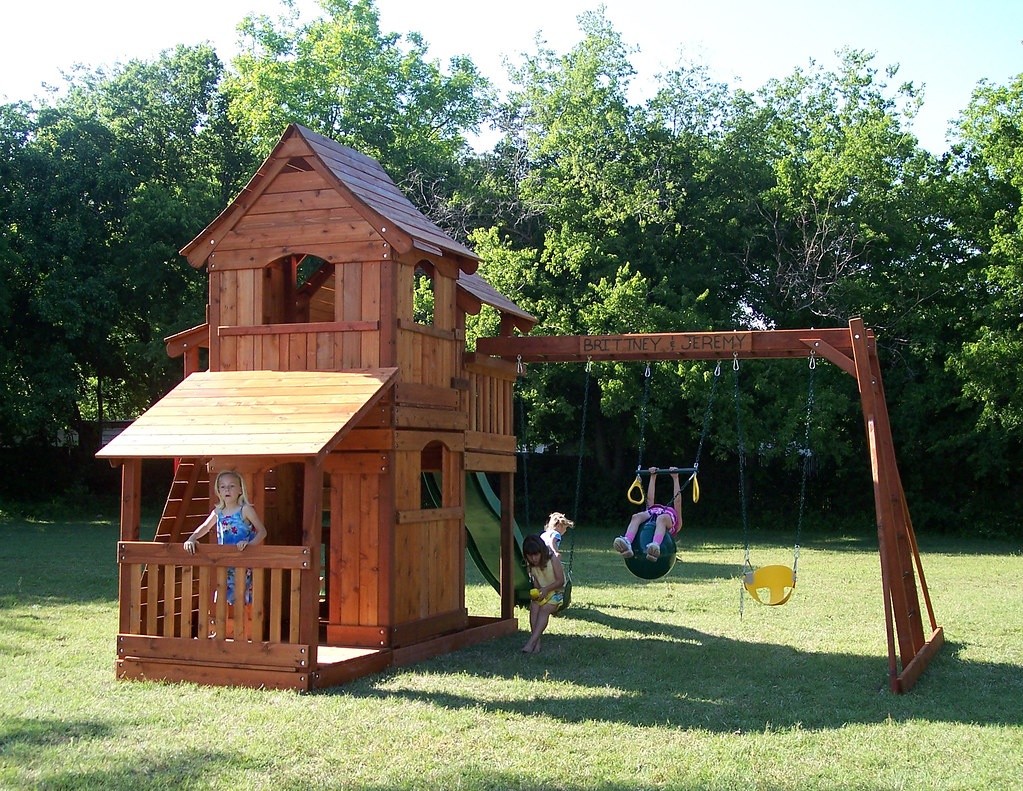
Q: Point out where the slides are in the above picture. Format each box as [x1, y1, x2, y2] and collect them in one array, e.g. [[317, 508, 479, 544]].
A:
[[421, 469, 532, 607]]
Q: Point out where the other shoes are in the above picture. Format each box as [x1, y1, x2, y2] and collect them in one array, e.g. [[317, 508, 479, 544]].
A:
[[645, 544, 660, 563], [613, 536, 634, 558]]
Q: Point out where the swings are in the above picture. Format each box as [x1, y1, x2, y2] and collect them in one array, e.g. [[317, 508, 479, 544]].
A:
[[623, 359, 722, 579], [517, 356, 593, 615], [733, 348, 817, 622]]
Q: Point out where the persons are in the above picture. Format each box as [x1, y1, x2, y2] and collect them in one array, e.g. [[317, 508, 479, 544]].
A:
[[614, 467, 683, 562], [183, 470, 267, 639], [521, 535, 565, 654], [540, 512, 573, 557]]
